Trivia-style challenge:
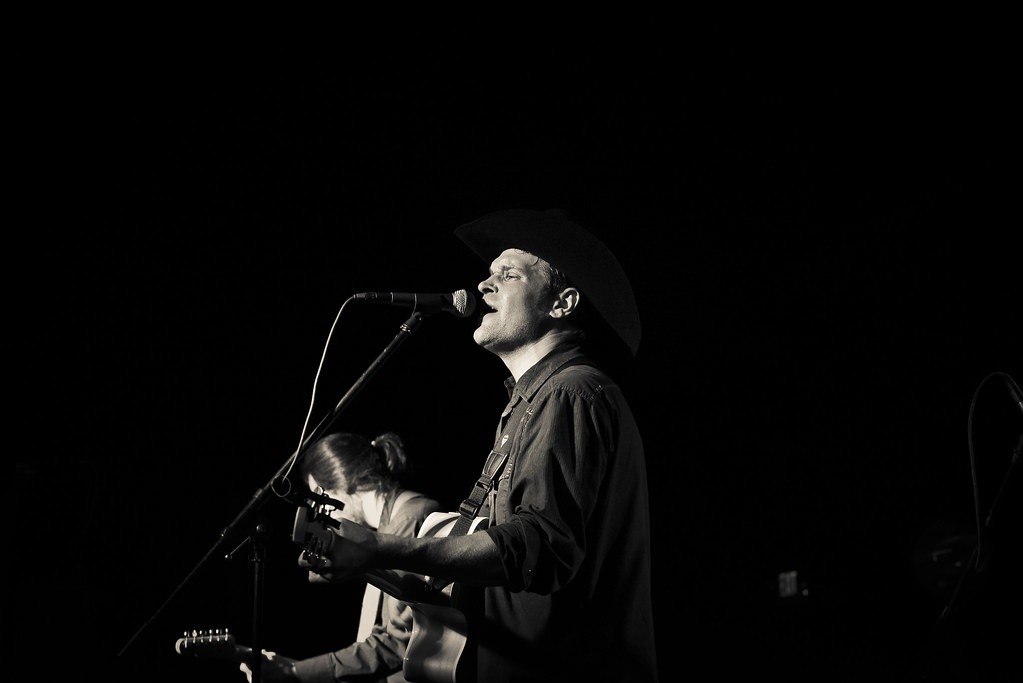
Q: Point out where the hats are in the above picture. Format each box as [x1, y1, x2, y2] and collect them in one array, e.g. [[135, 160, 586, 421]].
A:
[[453, 208, 642, 359]]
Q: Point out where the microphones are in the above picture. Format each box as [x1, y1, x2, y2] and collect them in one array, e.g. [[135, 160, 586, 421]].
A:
[[227, 524, 266, 560], [1002, 373, 1023, 415], [357, 288, 479, 320]]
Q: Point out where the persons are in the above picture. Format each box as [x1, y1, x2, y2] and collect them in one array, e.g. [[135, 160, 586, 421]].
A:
[[231, 423, 475, 682], [223, 219, 657, 680]]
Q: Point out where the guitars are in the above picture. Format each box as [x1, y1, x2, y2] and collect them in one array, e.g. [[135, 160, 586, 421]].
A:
[[289, 487, 479, 683], [175, 629, 297, 683]]
